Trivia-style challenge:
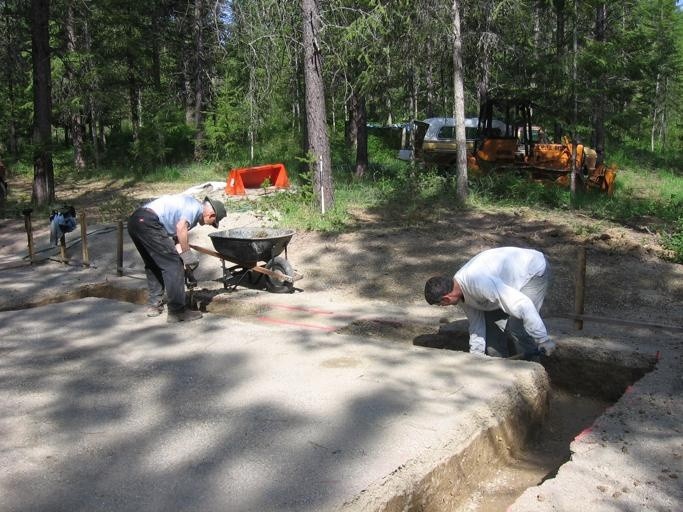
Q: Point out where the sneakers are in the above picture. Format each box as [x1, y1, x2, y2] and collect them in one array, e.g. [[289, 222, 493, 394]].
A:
[[146, 304, 164, 317], [166, 308, 202, 323]]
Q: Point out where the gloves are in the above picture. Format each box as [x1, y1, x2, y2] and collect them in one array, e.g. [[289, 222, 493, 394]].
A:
[[537, 340, 558, 358], [179, 249, 199, 291]]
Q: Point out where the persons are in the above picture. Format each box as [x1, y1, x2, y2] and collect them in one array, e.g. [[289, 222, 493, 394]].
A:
[[422, 246, 558, 364], [126, 194, 228, 323]]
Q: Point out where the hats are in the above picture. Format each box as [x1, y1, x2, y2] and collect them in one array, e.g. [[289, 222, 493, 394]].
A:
[[202, 196, 227, 229]]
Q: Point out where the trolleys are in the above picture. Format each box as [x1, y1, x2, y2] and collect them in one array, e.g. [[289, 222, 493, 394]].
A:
[[173, 225, 305, 292]]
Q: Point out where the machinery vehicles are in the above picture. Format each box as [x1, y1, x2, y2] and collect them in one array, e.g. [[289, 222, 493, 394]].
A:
[[466, 87, 619, 197]]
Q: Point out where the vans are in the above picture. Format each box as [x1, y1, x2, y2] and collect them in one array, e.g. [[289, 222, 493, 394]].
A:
[[412, 117, 548, 173]]
